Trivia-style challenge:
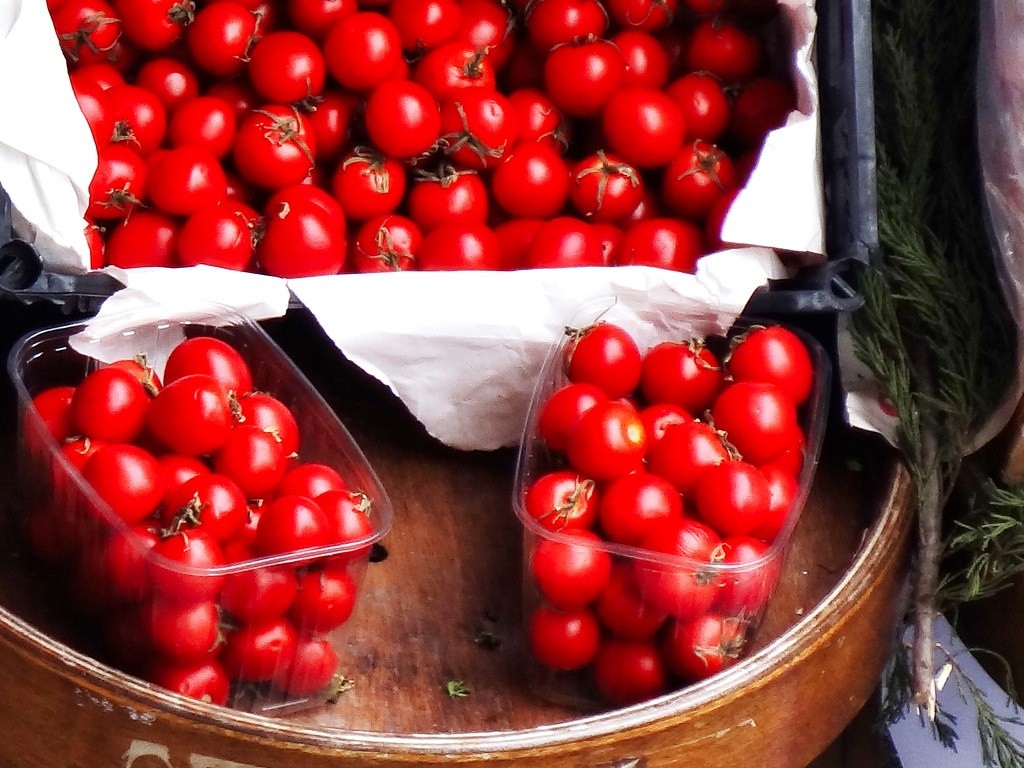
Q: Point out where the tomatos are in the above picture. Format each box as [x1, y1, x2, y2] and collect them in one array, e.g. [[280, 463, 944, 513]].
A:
[[23, 336, 376, 714], [44, 0, 793, 274], [522, 322, 814, 704]]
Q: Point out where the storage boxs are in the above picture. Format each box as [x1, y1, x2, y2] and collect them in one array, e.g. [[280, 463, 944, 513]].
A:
[[0, 0, 881, 449]]
